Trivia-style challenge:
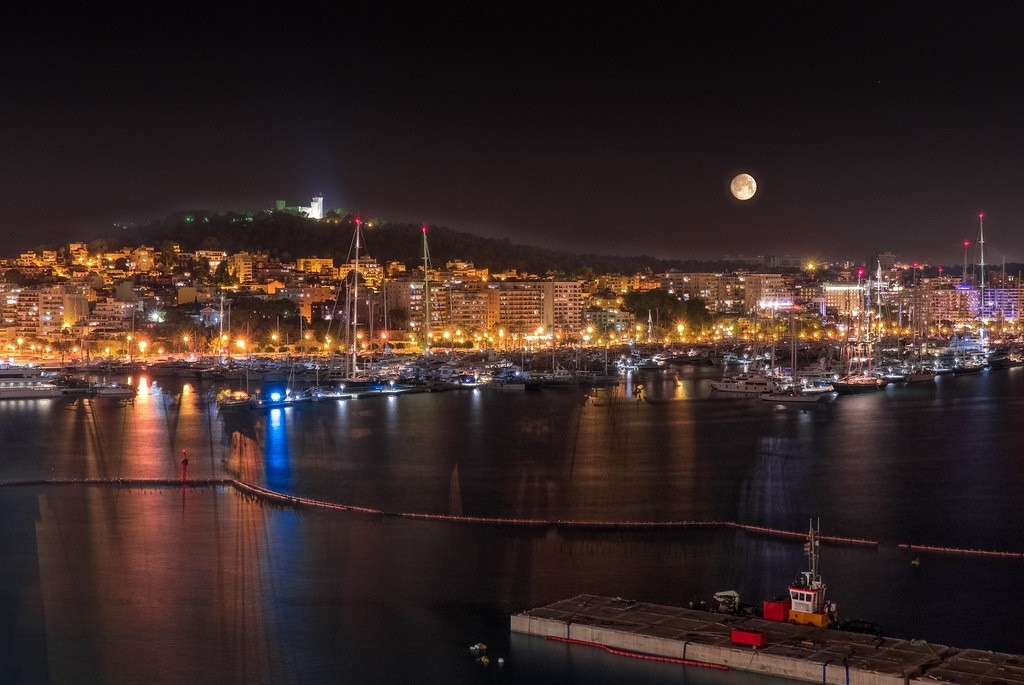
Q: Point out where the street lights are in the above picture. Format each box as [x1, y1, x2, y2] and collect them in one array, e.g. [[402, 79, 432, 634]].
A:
[[9, 320, 749, 365]]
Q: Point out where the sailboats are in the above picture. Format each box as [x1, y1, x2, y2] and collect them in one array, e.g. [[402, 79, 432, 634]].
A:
[[763, 312, 840, 404], [834, 274, 887, 394], [948, 240, 988, 377], [893, 261, 938, 383]]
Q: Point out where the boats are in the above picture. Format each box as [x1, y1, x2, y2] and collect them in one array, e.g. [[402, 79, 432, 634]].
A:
[[788, 517, 885, 637], [707, 377, 774, 394], [0, 381, 66, 398], [220, 388, 251, 407], [1, 208, 1024, 405]]
